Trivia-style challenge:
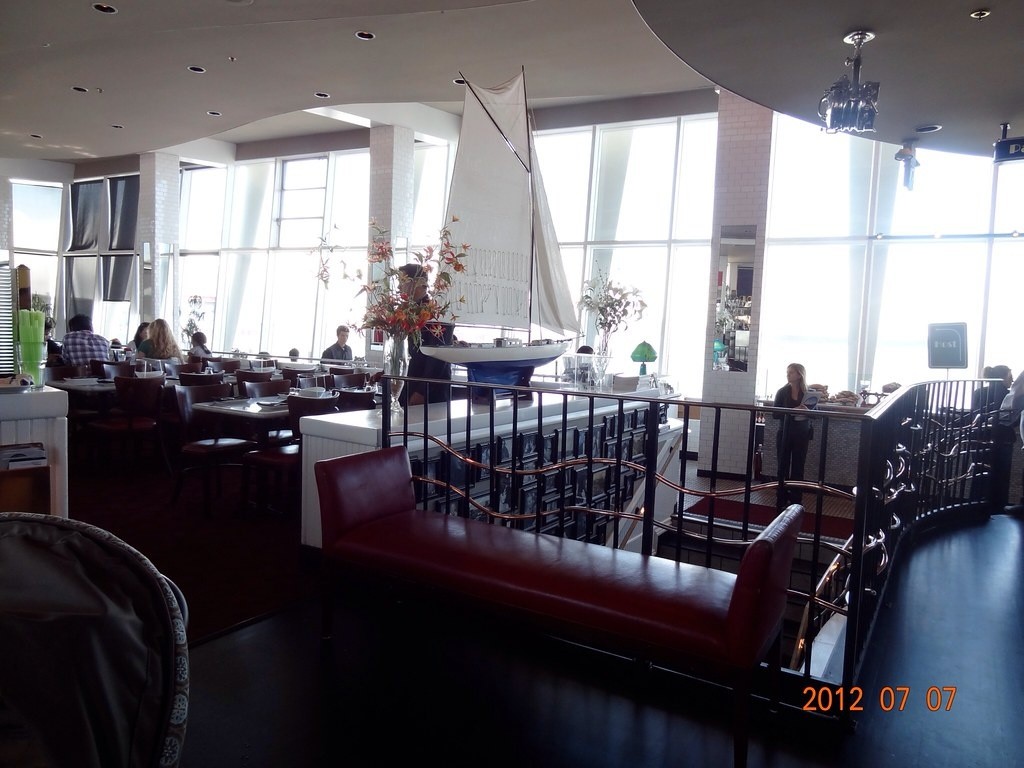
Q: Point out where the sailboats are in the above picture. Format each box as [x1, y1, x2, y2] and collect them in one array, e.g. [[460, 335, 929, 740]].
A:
[[420, 64, 588, 396]]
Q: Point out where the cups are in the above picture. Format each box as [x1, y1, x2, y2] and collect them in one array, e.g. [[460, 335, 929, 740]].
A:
[[13, 339, 48, 389]]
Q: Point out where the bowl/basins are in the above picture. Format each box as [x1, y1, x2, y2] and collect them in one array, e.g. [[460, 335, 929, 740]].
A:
[[64, 358, 385, 403]]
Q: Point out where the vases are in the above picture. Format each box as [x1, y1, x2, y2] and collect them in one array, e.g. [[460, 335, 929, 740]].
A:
[[377, 330, 414, 416], [595, 328, 618, 390]]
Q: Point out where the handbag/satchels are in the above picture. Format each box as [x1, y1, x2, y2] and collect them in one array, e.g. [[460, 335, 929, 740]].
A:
[[808, 426, 814, 440]]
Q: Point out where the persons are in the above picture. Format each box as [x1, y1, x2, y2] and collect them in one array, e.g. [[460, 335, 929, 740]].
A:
[[771, 362, 815, 506], [127, 321, 152, 353], [62, 314, 111, 368], [392, 263, 452, 407], [188, 331, 212, 363], [136, 318, 186, 364], [563, 345, 599, 387], [289, 349, 299, 363], [973, 370, 1024, 505], [320, 325, 352, 365], [970, 365, 1014, 512], [41, 316, 61, 366]]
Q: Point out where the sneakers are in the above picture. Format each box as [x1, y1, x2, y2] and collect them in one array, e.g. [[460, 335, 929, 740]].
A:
[[1003, 504, 1024, 516]]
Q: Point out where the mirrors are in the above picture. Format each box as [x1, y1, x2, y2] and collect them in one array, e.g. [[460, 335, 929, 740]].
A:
[[712, 223, 759, 372]]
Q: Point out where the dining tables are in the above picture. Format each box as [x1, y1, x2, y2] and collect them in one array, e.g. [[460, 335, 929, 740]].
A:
[[116, 353, 252, 369], [47, 366, 383, 410], [192, 383, 384, 522]]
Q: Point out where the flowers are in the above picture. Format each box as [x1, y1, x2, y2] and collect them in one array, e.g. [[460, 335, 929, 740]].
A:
[[314, 211, 468, 349], [576, 258, 648, 337]]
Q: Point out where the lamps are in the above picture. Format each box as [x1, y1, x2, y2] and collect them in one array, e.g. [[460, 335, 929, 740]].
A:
[[628, 341, 658, 376], [818, 26, 886, 136]]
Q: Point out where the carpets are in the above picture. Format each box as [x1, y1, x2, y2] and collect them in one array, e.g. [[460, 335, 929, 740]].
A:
[[681, 494, 856, 545]]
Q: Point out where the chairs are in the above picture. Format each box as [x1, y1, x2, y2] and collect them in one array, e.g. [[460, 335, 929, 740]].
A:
[[255, 390, 340, 513], [103, 364, 145, 380], [248, 359, 278, 371], [298, 374, 336, 394], [84, 374, 167, 479], [175, 379, 261, 516], [89, 360, 126, 376], [242, 380, 299, 481], [328, 368, 357, 377], [236, 370, 274, 396], [282, 368, 316, 389], [163, 363, 203, 376], [331, 388, 378, 412], [333, 370, 368, 389], [186, 355, 223, 370], [206, 360, 241, 375], [178, 372, 225, 386]]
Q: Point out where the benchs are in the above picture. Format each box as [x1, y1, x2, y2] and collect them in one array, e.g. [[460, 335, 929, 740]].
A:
[[312, 444, 808, 768]]
